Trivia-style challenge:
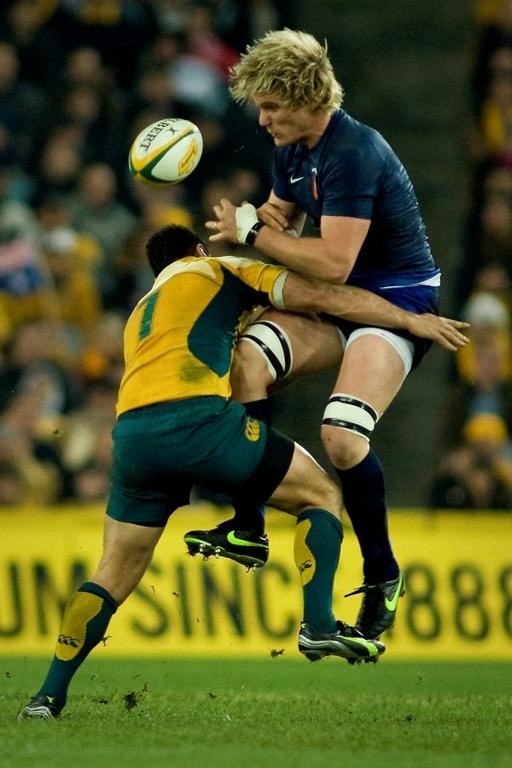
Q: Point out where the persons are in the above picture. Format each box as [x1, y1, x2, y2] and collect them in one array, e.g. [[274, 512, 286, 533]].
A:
[[176, 20, 443, 640], [12, 218, 475, 720], [1, 1, 512, 515]]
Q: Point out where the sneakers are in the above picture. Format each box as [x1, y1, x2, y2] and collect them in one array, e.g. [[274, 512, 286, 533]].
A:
[[296, 618, 388, 664], [15, 695, 66, 721], [181, 523, 270, 574], [353, 569, 406, 640]]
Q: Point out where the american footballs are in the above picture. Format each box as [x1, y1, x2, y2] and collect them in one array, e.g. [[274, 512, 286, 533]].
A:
[[128, 118, 204, 188]]
